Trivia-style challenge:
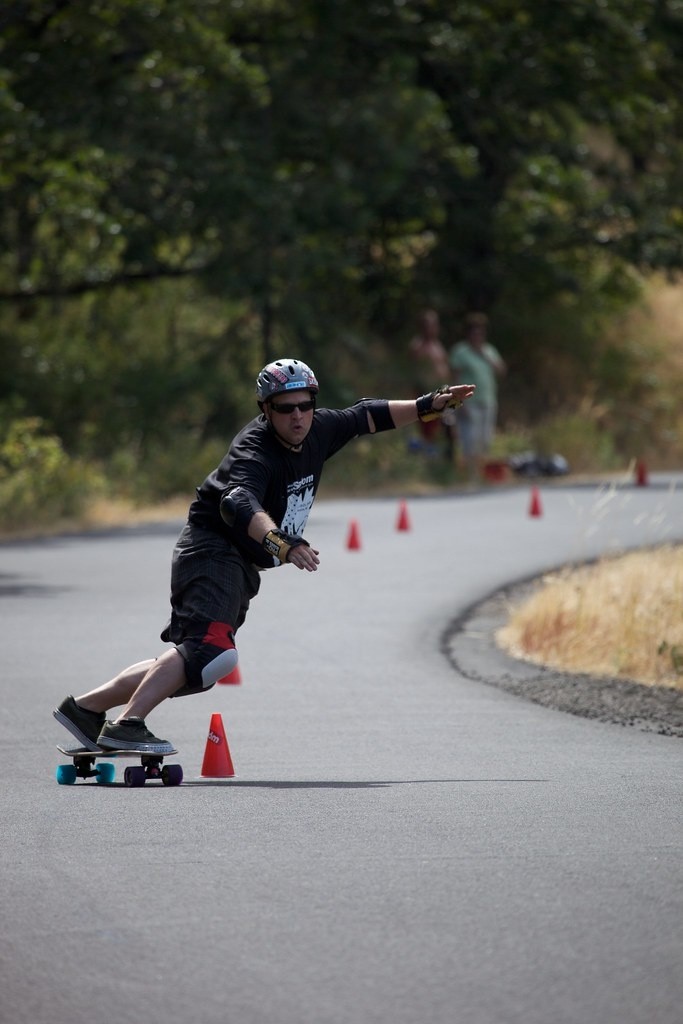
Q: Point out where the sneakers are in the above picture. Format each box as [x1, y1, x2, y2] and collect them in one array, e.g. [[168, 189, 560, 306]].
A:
[[53, 695, 106, 751], [97, 716, 173, 753]]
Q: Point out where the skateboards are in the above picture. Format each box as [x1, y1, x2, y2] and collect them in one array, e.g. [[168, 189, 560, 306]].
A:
[[53, 742, 184, 788]]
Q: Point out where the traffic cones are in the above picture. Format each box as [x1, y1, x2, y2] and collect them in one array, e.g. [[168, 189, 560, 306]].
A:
[[395, 501, 409, 533], [212, 660, 241, 684], [347, 517, 361, 550], [638, 461, 650, 488], [529, 486, 544, 520], [200, 711, 239, 779]]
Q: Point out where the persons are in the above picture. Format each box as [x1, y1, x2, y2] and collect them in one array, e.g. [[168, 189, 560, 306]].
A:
[[408, 309, 505, 480], [50, 357, 476, 751]]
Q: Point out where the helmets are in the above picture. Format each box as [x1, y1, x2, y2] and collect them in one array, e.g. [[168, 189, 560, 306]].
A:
[[255, 359, 319, 402]]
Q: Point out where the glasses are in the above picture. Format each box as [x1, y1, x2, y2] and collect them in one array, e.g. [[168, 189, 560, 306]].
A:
[[265, 397, 315, 413]]
